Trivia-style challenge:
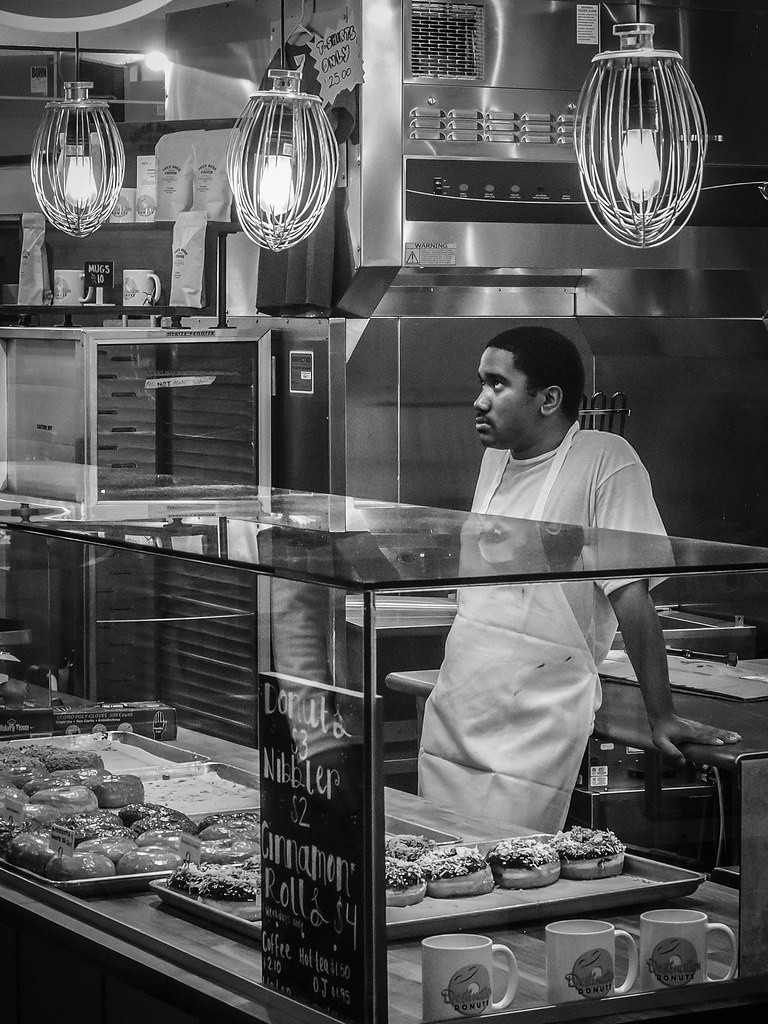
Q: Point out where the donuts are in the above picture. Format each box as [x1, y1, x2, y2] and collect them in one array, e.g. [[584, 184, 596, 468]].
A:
[[167, 826, 625, 920], [0, 744, 261, 881]]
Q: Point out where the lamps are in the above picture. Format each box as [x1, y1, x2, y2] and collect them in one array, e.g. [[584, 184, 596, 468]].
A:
[[225, 0, 341, 255], [572, 0, 709, 252], [30, 30, 127, 239]]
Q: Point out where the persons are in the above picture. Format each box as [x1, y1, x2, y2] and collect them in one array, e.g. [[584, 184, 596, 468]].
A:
[[267, 577, 358, 832], [413, 326, 740, 838]]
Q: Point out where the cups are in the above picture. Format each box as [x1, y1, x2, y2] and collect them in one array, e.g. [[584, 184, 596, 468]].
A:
[[123, 269, 161, 306], [640, 910, 739, 988], [53, 269, 93, 305], [544, 919, 638, 1003], [421, 934, 519, 1020]]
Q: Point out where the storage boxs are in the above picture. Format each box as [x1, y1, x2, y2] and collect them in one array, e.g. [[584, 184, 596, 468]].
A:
[[1, 699, 178, 741]]
